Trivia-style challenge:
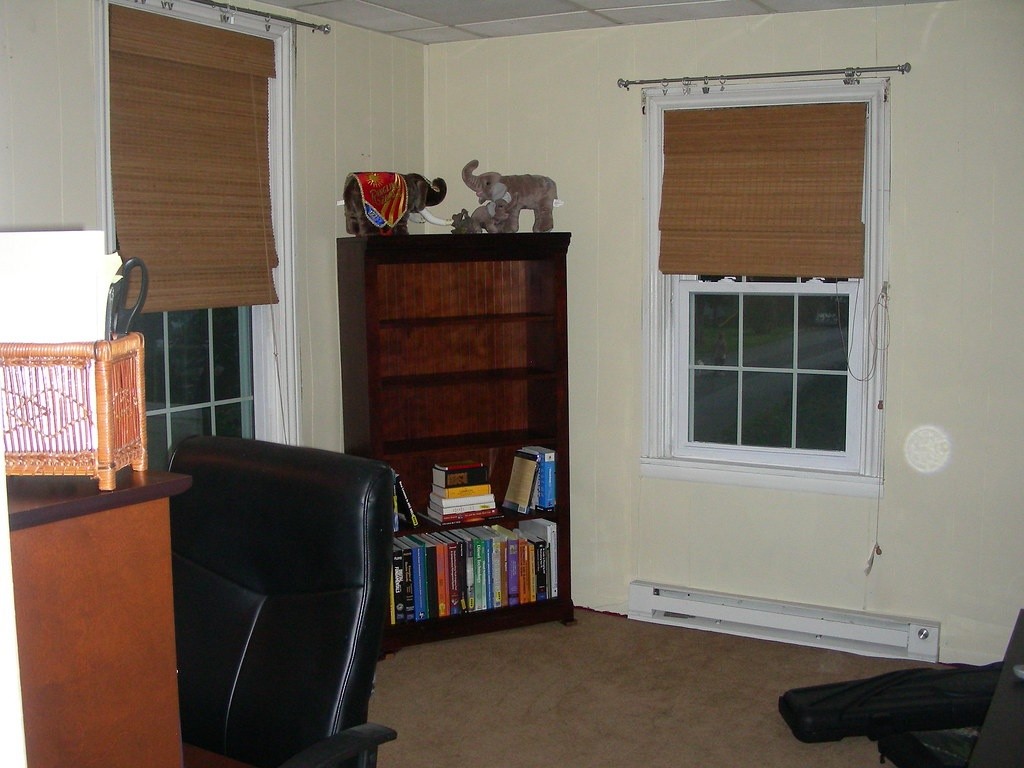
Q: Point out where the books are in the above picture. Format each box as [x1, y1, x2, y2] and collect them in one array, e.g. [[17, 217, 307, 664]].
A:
[[392, 445, 559, 625]]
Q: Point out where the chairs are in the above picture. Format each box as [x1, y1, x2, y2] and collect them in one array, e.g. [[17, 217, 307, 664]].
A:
[[169, 435, 397, 768]]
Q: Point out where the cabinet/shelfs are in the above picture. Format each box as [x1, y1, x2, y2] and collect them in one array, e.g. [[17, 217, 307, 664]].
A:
[[4, 469, 191, 768], [335, 232, 578, 660]]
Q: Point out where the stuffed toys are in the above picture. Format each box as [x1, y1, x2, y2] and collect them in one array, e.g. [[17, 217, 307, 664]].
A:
[[345, 159, 556, 233]]
